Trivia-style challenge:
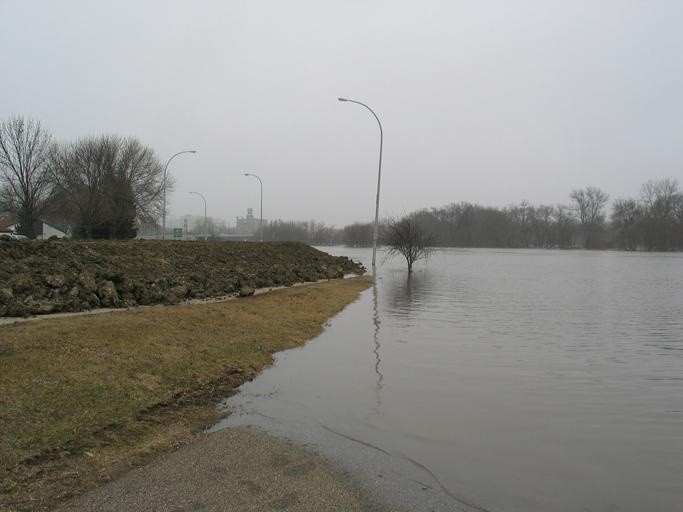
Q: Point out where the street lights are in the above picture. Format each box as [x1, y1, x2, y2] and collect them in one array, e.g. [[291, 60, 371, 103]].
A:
[[339, 97, 383, 268], [189, 192, 207, 241], [162, 150, 198, 239], [245, 174, 263, 242]]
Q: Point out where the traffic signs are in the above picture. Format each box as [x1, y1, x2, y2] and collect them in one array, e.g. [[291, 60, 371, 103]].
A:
[[173, 228, 182, 238]]
